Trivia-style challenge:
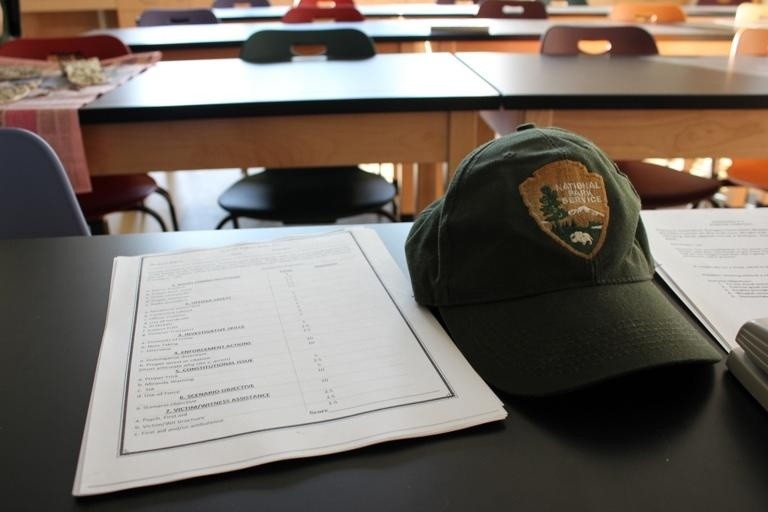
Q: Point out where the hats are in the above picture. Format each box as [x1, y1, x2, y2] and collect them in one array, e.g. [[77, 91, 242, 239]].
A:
[[403, 121, 723, 400]]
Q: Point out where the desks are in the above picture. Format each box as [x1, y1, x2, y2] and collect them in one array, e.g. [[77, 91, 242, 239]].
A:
[[2, 47, 766, 221], [70, 16, 767, 55], [1, 221, 768, 511]]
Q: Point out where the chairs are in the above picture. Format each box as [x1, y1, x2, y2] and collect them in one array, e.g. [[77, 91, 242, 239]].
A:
[[604, 3, 688, 25], [283, 6, 365, 23], [474, 1, 552, 21], [530, 21, 722, 215], [210, 26, 403, 231], [0, 32, 180, 243], [134, 5, 218, 26], [4, 120, 93, 239]]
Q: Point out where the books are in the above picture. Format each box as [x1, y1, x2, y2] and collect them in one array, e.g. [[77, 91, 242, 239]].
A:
[[637, 208, 768, 355]]
